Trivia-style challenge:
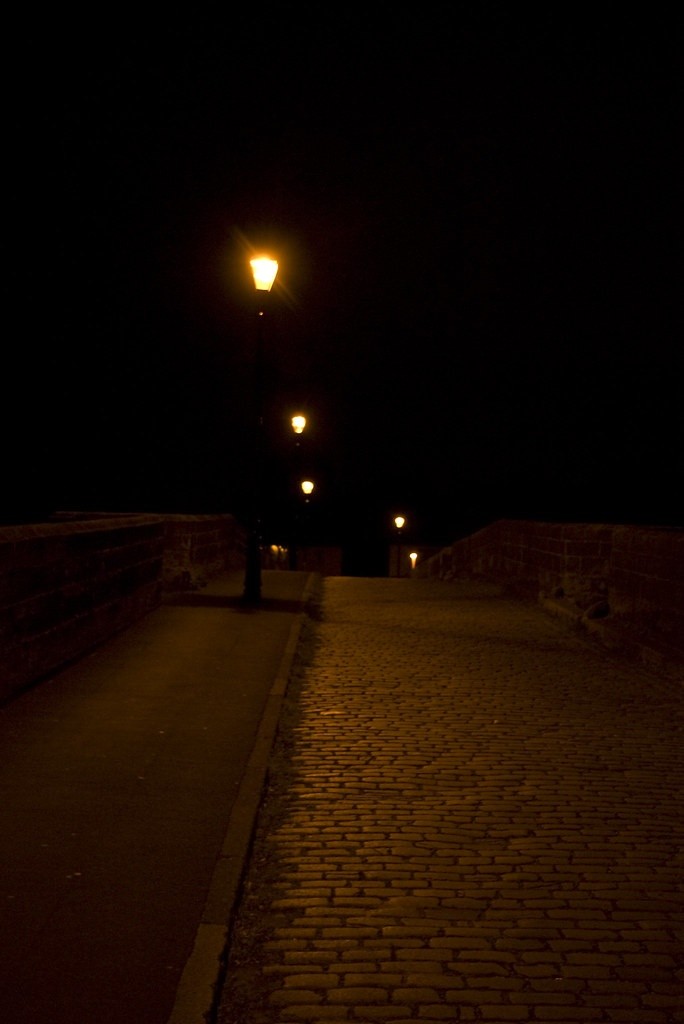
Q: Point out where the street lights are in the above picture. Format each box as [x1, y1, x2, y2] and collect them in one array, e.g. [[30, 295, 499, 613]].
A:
[[231, 244, 285, 603]]
